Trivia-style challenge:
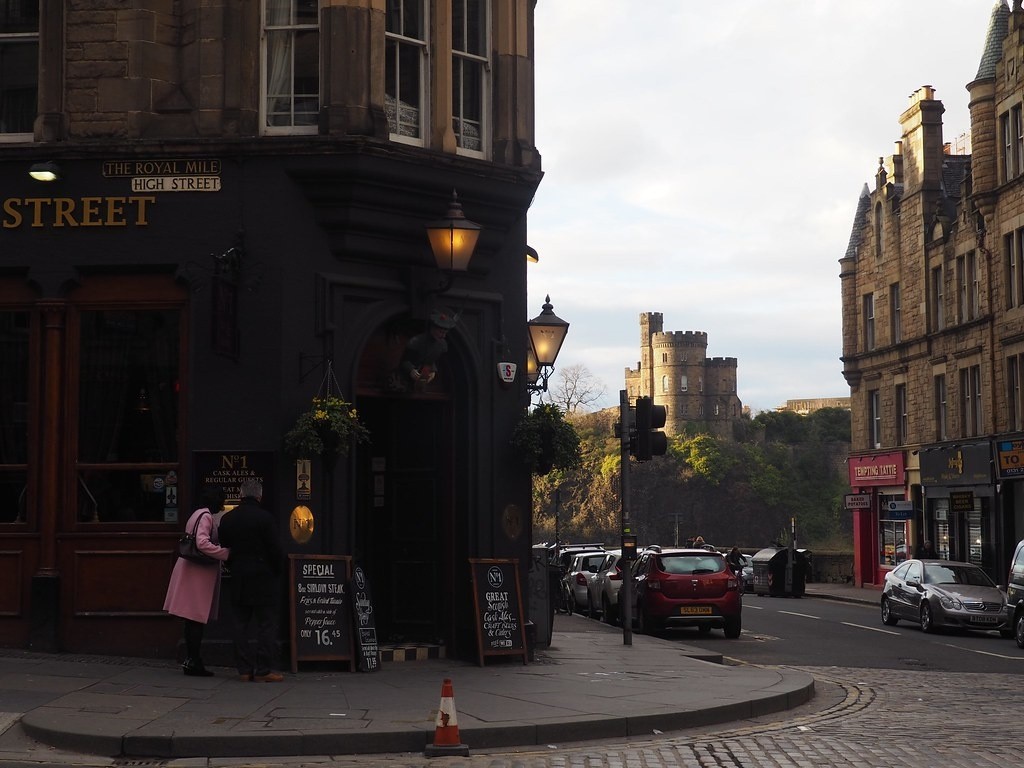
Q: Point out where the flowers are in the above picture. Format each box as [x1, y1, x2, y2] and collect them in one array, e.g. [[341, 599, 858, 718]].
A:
[[282, 397, 375, 457]]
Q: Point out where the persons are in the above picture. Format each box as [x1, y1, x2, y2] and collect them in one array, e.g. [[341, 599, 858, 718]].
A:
[[162, 486, 231, 677], [915, 540, 940, 559], [218, 480, 290, 682], [684, 535, 707, 549], [726, 546, 748, 579]]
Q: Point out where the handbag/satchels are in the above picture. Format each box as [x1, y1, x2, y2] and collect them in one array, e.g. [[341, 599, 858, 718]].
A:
[[175, 510, 219, 566]]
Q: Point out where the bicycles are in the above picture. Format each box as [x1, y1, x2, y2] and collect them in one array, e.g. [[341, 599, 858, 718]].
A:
[[548, 554, 573, 615], [734, 564, 747, 596]]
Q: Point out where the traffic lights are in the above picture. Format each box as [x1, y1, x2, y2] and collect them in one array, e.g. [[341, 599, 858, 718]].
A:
[[633, 396, 667, 462]]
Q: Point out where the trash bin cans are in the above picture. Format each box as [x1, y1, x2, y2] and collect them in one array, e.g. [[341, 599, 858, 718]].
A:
[[751, 546, 808, 596]]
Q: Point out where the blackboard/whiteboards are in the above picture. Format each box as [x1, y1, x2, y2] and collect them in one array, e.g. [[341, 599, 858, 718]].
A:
[[345, 560, 380, 674], [468, 557, 526, 655], [287, 553, 354, 662]]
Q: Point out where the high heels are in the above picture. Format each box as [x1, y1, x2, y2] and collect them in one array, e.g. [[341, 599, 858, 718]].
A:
[[181, 655, 215, 677]]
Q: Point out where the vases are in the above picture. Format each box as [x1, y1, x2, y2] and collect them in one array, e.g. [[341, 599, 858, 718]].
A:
[[309, 422, 339, 451]]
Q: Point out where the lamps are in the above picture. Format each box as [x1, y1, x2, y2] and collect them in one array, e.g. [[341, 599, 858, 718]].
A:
[[526, 292, 571, 390], [418, 185, 487, 293], [26, 157, 64, 185]]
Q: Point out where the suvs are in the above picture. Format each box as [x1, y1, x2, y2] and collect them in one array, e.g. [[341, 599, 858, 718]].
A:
[[1006, 540, 1024, 648], [536, 542, 741, 639]]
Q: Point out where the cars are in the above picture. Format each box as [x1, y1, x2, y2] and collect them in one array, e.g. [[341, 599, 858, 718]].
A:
[[695, 554, 754, 592], [880, 558, 1007, 634]]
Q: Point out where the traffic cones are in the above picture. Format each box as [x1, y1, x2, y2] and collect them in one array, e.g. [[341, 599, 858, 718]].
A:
[[423, 680, 470, 758]]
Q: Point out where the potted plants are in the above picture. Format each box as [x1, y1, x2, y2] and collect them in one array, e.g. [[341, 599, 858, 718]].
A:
[[501, 400, 585, 479]]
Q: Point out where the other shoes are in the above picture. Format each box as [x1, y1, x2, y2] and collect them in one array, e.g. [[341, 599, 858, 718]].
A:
[[237, 673, 252, 681], [254, 673, 284, 684]]
